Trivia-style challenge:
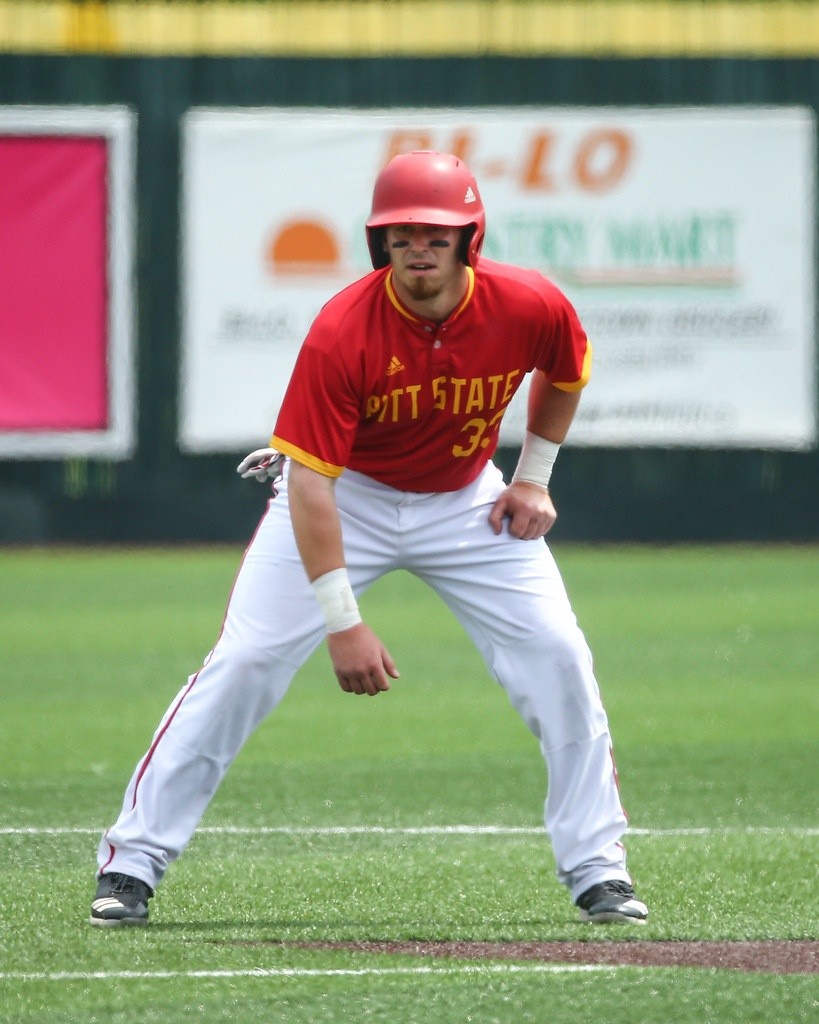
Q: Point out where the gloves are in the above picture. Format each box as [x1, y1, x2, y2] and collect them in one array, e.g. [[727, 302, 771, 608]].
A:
[[237, 448, 285, 483]]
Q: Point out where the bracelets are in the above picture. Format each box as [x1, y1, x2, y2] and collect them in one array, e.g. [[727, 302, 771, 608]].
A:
[[512, 431, 561, 489], [310, 568, 363, 633]]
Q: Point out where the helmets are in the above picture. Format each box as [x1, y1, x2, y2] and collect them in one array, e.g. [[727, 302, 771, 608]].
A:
[[366, 152, 486, 270]]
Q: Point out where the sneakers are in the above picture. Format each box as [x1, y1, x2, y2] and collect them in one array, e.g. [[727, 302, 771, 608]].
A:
[[89, 872, 154, 927], [575, 880, 648, 925]]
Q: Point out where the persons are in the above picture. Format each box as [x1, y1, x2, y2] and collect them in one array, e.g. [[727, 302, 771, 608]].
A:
[[90, 148, 650, 925]]
